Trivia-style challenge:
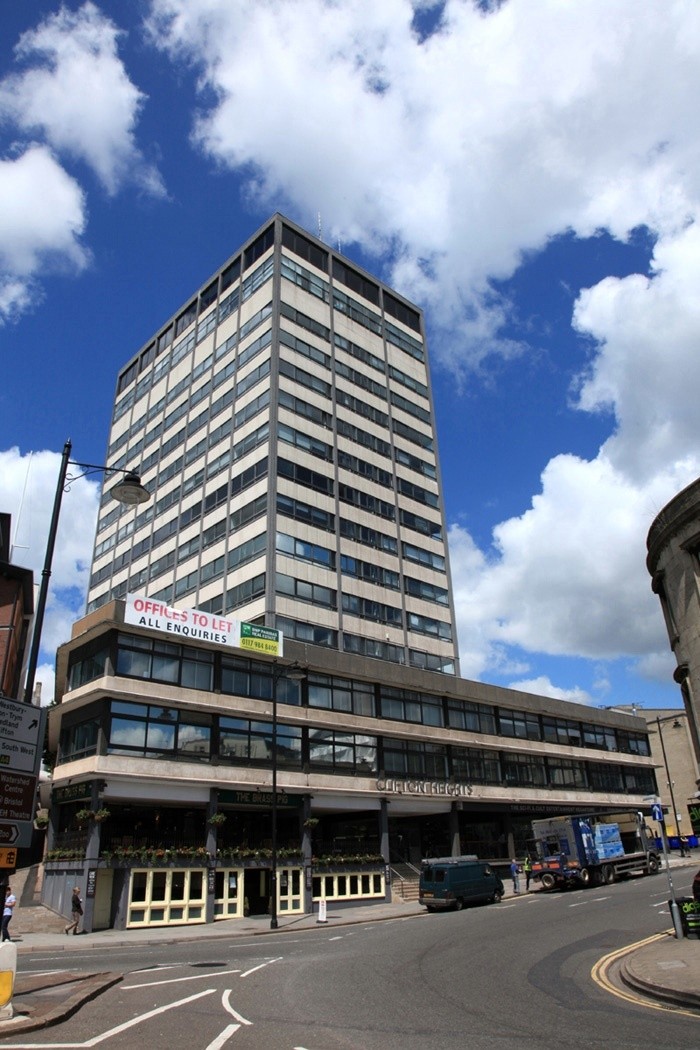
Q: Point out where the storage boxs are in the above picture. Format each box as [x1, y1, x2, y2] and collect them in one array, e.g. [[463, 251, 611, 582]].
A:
[[591, 822, 625, 859]]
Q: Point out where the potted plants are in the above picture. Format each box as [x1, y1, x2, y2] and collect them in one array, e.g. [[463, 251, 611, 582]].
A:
[[313, 853, 385, 868], [75, 808, 111, 826]]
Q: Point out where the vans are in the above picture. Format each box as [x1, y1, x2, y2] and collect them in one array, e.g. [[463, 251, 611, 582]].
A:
[[417, 861, 505, 910]]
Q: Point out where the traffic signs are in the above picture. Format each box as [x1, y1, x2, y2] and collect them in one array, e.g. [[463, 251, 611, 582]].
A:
[[0, 697, 46, 777], [1, 820, 21, 848]]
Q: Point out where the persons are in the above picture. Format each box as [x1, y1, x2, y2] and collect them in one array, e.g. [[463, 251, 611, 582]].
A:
[[64, 887, 83, 935], [1, 888, 17, 942], [680, 832, 691, 857], [524, 854, 532, 893], [559, 852, 568, 868], [510, 858, 522, 894]]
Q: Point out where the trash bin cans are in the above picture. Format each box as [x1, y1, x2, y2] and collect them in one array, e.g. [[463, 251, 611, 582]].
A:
[[669, 897, 696, 937]]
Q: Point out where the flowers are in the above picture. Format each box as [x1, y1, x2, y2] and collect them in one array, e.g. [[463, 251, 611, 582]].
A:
[[103, 846, 305, 865]]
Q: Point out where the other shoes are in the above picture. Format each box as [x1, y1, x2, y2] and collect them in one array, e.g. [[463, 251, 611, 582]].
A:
[[73, 932, 81, 935], [513, 891, 521, 896], [525, 890, 531, 893], [65, 925, 69, 935]]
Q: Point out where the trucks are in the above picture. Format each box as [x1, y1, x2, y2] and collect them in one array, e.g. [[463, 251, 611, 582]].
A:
[[525, 809, 662, 891]]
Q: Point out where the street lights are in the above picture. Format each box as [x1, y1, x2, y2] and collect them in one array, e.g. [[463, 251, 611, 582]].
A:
[[656, 715, 685, 859], [271, 659, 306, 925], [0, 439, 150, 925]]
